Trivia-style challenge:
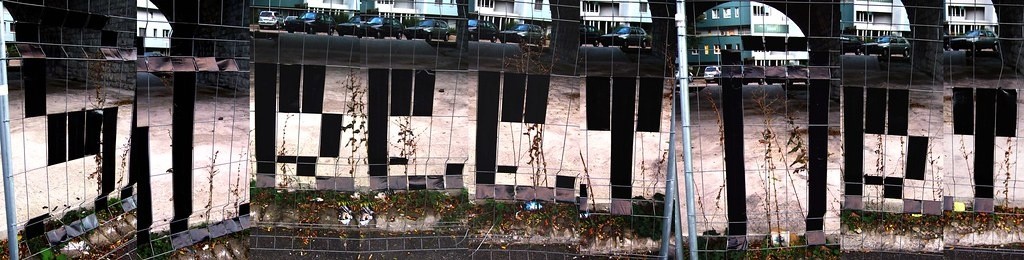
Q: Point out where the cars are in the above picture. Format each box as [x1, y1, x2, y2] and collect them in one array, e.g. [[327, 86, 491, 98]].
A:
[[859, 35, 913, 60], [950, 30, 998, 52], [841, 34, 866, 56], [579, 25, 600, 48], [404, 19, 450, 42], [600, 26, 651, 50], [284, 16, 307, 33], [335, 15, 365, 36], [703, 66, 721, 85], [468, 20, 500, 44], [499, 23, 547, 46]]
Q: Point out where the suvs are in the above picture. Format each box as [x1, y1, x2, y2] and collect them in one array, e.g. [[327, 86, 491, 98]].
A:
[[296, 12, 337, 36], [258, 10, 284, 30], [357, 17, 404, 40]]
